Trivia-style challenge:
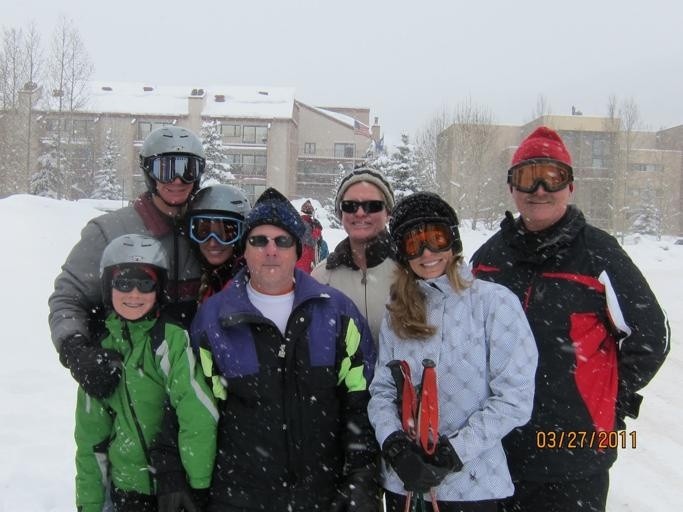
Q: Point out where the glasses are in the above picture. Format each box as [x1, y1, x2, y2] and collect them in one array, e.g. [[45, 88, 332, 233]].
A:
[[507, 157, 573, 192], [189, 215, 241, 245], [341, 200, 384, 213], [145, 154, 204, 183], [112, 279, 154, 292], [248, 236, 296, 247], [396, 222, 454, 259]]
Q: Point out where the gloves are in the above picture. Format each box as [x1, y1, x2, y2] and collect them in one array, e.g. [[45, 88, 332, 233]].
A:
[[155, 469, 197, 512], [58, 333, 122, 396], [331, 475, 383, 512], [382, 430, 463, 492]]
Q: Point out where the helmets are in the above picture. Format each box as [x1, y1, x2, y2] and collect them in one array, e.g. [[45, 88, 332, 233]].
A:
[[187, 185, 250, 250], [100, 234, 170, 278], [139, 127, 206, 191]]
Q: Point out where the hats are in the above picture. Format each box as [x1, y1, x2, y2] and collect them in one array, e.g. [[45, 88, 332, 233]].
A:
[[512, 127, 574, 191], [247, 187, 305, 238], [334, 167, 395, 214], [391, 193, 459, 245]]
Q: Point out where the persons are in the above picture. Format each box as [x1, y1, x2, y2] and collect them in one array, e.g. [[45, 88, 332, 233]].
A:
[[181, 183, 250, 315], [468, 125, 672, 510], [309, 160, 409, 350], [314, 233, 330, 262], [74, 233, 222, 512], [46, 124, 208, 400], [295, 199, 323, 275], [364, 189, 543, 511], [188, 186, 379, 510]]
